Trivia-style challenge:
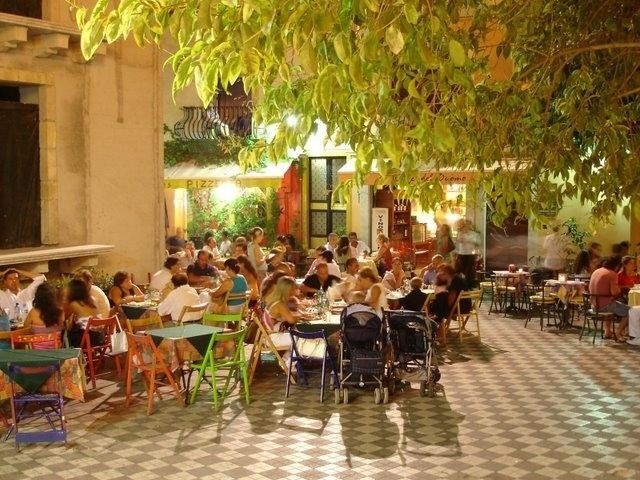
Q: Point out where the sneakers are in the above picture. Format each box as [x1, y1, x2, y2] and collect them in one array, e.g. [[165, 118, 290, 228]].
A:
[[277, 359, 298, 384]]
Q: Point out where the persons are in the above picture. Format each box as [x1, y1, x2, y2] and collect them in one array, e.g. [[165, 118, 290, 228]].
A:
[[0, 221, 481, 374], [543, 224, 640, 342]]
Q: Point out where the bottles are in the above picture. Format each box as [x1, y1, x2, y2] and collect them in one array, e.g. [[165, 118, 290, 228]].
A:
[[3, 302, 28, 324], [393, 199, 409, 238]]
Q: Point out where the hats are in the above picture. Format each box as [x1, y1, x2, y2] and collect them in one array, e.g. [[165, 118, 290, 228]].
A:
[[235, 236, 247, 245], [621, 255, 638, 263], [270, 248, 284, 257]]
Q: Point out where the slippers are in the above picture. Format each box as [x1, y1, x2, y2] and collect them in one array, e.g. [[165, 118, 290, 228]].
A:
[[604, 334, 616, 339], [615, 334, 635, 343]]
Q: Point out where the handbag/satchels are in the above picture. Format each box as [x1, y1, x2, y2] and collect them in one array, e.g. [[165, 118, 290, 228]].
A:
[[101, 315, 128, 358], [293, 335, 327, 360]]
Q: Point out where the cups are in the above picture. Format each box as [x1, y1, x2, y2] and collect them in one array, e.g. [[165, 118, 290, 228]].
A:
[[301, 284, 346, 318], [135, 288, 162, 307], [389, 279, 435, 296]]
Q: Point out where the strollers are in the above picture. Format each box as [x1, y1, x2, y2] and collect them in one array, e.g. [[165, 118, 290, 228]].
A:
[[378, 303, 445, 399], [326, 301, 392, 406]]
